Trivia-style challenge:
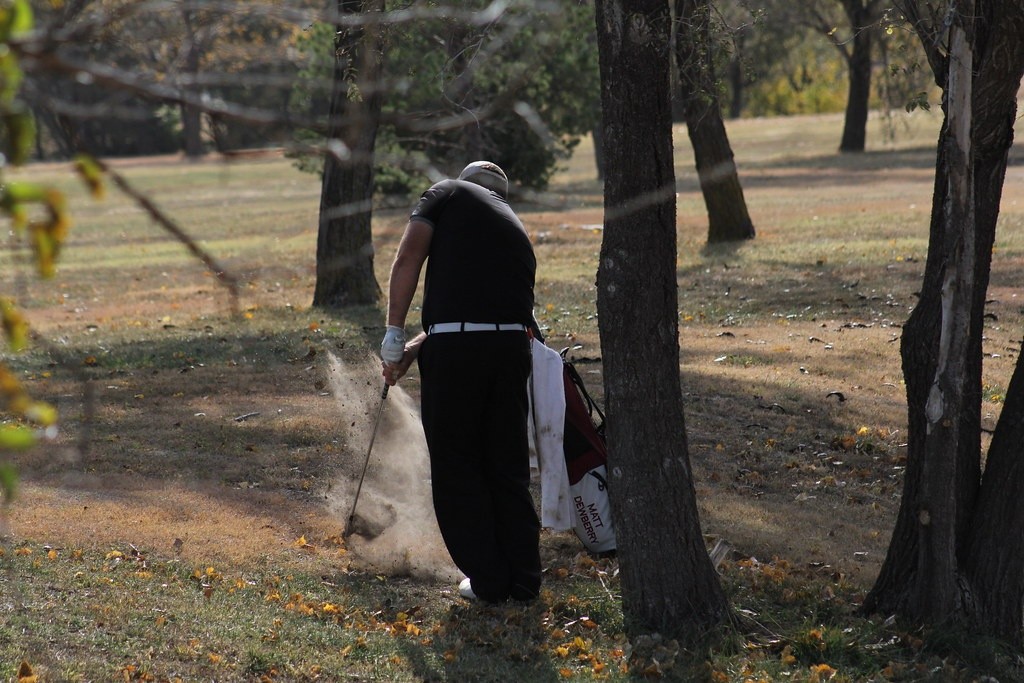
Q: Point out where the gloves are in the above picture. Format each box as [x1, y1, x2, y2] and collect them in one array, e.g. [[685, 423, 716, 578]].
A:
[[381, 325, 406, 366]]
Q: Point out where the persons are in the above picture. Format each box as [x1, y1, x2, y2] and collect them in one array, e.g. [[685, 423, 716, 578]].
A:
[[379, 159, 544, 607]]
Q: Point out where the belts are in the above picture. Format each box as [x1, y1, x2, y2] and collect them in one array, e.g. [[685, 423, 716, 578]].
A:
[[428, 322, 528, 335]]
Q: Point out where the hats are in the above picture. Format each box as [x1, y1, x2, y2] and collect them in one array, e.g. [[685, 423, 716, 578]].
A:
[[457, 161, 508, 182]]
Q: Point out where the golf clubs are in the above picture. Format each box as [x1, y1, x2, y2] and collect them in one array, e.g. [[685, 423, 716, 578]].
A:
[[560, 346, 570, 361], [344, 384, 388, 538]]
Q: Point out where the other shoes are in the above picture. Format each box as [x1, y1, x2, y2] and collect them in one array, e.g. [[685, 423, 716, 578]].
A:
[[458, 578, 477, 600]]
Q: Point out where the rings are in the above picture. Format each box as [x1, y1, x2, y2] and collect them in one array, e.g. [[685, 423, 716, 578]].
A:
[[392, 370, 399, 375], [393, 375, 398, 380]]
